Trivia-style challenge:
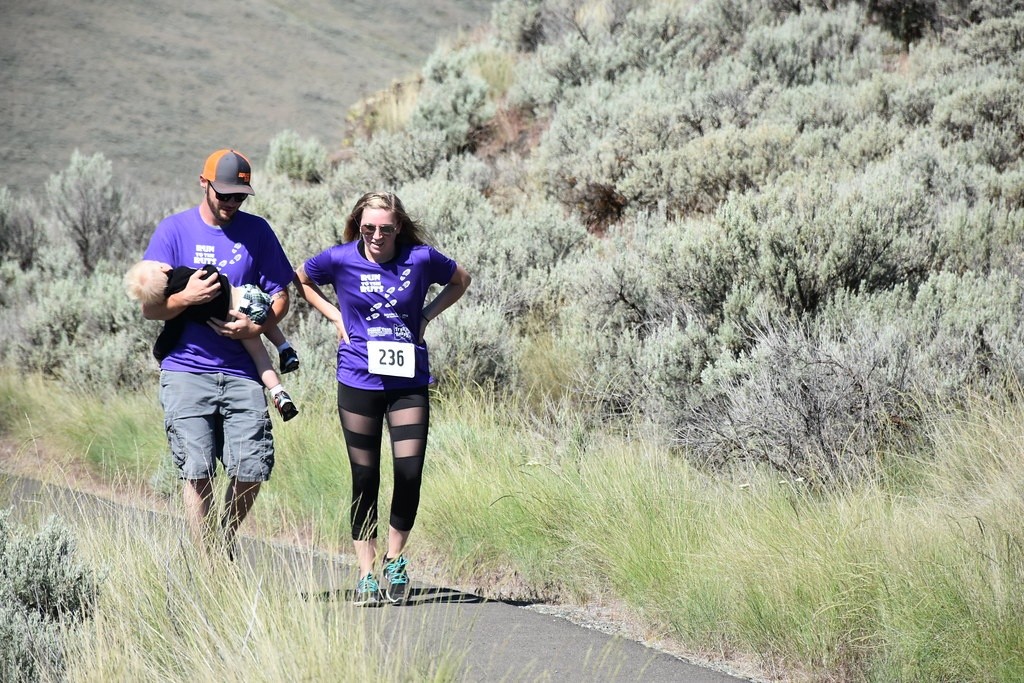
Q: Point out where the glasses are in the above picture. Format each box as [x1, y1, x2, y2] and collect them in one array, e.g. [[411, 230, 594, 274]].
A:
[[208, 180, 248, 202], [360, 224, 401, 236]]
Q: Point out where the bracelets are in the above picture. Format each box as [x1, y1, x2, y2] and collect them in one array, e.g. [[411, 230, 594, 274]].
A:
[[422, 313, 430, 323]]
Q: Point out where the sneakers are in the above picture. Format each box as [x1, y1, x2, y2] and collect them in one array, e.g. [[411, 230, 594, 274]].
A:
[[274, 391, 299, 421], [279, 347, 299, 374], [356, 573, 381, 606], [381, 551, 414, 605]]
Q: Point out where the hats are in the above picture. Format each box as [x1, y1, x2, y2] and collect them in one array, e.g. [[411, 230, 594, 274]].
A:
[[203, 149, 255, 196]]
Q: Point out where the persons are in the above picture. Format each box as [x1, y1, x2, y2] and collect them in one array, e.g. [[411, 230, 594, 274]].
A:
[[122, 259, 300, 422], [295, 191, 471, 608], [139, 147, 296, 562]]
